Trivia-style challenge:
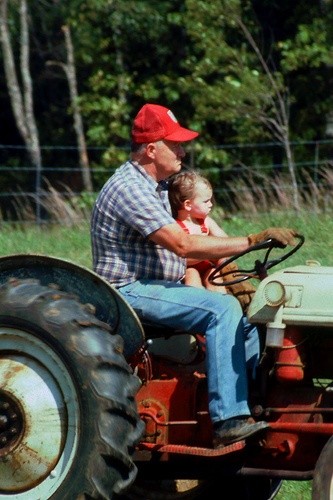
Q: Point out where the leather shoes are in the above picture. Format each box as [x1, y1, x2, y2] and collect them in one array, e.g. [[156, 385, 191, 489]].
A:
[[213, 417, 269, 449]]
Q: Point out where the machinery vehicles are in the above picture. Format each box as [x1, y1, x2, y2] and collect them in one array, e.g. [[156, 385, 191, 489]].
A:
[[0, 230, 333, 499]]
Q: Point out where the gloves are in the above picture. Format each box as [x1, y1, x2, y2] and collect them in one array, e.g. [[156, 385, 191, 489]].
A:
[[225, 266, 257, 303], [250, 225, 296, 247]]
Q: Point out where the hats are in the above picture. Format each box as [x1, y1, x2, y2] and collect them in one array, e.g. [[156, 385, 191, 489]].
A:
[[130, 104, 199, 142]]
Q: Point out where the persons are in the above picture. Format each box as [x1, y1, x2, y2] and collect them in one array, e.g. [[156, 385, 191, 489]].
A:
[[166, 170, 234, 296], [90, 104, 295, 448]]
